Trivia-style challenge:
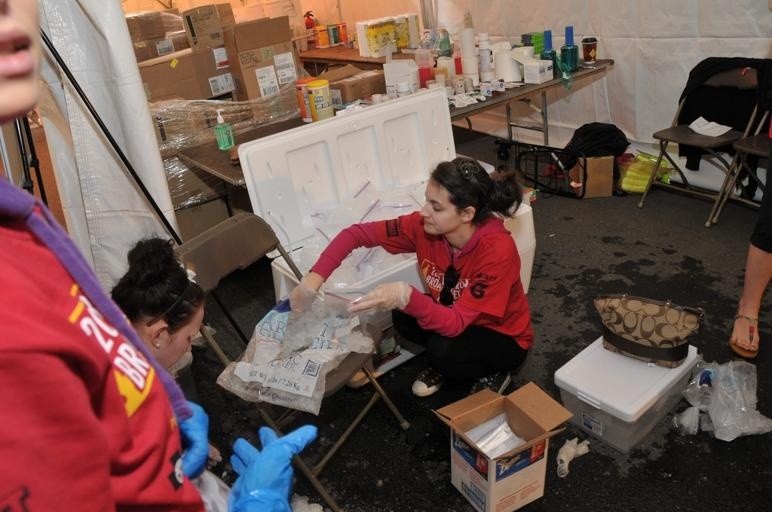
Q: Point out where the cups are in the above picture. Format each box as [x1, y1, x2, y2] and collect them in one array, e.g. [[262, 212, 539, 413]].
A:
[[582, 38, 598, 63]]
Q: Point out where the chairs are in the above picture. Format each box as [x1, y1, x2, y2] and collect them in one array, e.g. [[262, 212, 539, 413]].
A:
[[173, 212, 413, 511], [635, 55, 772, 226]]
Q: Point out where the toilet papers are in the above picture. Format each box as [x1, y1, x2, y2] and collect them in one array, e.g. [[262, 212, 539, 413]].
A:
[[493, 48, 522, 82], [458, 29, 479, 85]]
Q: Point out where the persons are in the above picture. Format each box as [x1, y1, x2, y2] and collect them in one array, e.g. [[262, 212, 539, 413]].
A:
[[0, 1, 319, 512], [110, 236, 222, 470], [729, 162, 770, 360], [293, 158, 534, 400]]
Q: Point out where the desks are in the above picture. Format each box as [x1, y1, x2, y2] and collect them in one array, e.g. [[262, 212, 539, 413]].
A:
[[179, 46, 615, 225]]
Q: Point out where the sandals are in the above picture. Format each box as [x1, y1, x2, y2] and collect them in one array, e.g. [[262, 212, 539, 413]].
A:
[[729, 308, 761, 360]]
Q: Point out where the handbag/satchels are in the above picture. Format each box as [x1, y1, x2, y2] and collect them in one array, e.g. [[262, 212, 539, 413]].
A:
[[592, 292, 705, 368]]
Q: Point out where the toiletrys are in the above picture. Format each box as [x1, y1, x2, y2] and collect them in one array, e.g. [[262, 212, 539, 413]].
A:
[[213, 109, 235, 150]]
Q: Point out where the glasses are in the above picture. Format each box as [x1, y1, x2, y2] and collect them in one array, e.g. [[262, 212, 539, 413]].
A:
[[440, 264, 460, 306]]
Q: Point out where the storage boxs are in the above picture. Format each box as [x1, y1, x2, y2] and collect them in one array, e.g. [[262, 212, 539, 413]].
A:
[[125, 1, 385, 149], [552, 332, 700, 454], [426, 382, 574, 511]]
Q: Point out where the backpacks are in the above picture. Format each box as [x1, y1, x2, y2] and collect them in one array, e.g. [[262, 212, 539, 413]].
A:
[[556, 122, 631, 180]]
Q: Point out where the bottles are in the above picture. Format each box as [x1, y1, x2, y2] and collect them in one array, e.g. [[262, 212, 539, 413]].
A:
[[415, 28, 492, 96], [295, 77, 334, 123], [312, 22, 349, 49], [373, 81, 411, 106], [541, 24, 579, 79]]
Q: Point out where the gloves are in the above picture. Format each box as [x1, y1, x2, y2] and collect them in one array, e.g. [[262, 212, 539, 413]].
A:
[[179, 397, 210, 481], [226, 422, 319, 512]]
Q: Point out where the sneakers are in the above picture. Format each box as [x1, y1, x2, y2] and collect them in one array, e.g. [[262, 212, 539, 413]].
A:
[[468, 364, 512, 396], [411, 364, 448, 397]]
